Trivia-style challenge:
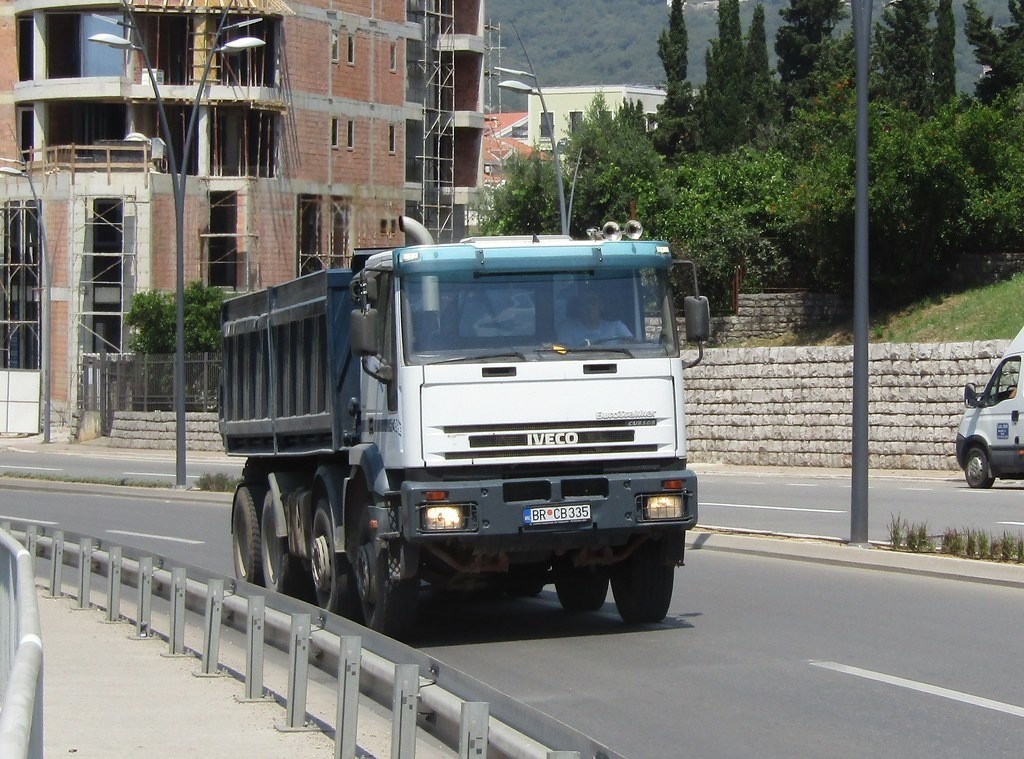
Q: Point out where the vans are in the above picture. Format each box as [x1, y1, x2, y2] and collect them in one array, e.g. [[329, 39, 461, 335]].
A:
[[957, 323, 1024, 488]]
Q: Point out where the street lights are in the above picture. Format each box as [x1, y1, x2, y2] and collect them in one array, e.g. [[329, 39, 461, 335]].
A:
[[86, 10, 268, 487], [0, 157, 53, 445], [496, 81, 571, 237]]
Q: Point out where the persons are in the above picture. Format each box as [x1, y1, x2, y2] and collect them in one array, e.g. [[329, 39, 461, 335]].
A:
[[556, 283, 634, 352]]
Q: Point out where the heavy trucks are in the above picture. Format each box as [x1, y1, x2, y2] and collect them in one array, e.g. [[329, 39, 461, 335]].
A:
[[217, 213, 711, 644]]
